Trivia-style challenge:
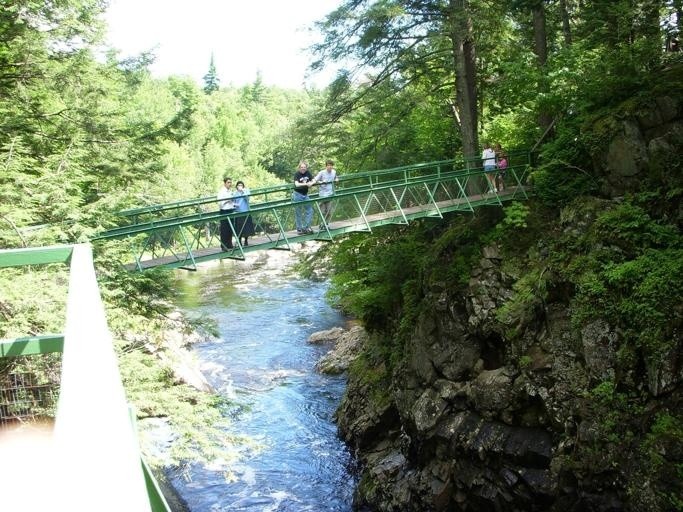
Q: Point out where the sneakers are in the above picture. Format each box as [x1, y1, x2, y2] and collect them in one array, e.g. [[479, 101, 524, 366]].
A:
[[487, 188, 505, 194], [296, 226, 332, 234], [222, 242, 248, 250]]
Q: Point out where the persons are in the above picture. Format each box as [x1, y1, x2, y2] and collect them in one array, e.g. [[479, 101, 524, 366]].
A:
[[290, 159, 338, 235], [219, 173, 254, 247], [481, 139, 508, 193]]
